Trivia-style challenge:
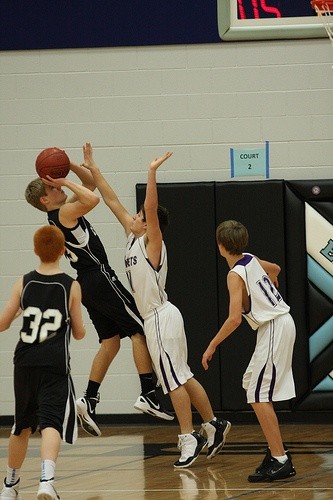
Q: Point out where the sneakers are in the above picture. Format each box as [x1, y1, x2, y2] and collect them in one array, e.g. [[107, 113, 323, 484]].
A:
[[134, 389, 174, 420], [202, 418, 232, 459], [0, 478, 20, 500], [173, 431, 208, 468], [248, 445, 296, 483], [38, 478, 59, 499], [75, 393, 101, 436]]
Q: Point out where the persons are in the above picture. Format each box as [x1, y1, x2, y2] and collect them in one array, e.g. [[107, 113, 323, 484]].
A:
[[25, 164, 174, 436], [0, 226, 84, 500], [202, 220, 296, 482], [80, 143, 230, 468]]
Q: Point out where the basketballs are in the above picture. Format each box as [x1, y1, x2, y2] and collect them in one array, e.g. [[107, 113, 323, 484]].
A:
[[36, 148, 71, 182]]
